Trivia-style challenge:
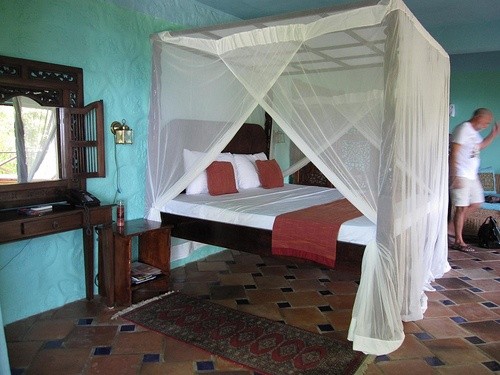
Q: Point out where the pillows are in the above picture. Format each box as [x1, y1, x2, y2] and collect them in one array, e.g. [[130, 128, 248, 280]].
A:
[[256, 159, 284, 189], [182, 149, 235, 194], [233, 153, 268, 190], [207, 161, 236, 196]]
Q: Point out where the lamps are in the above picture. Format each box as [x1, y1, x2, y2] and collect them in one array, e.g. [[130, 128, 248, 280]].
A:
[[112, 119, 135, 145], [273, 128, 285, 142]]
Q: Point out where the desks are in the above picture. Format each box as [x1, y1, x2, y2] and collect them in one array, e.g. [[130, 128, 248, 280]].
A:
[[0, 204, 118, 305]]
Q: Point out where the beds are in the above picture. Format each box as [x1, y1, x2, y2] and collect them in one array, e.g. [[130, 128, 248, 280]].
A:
[[146, 2, 453, 355]]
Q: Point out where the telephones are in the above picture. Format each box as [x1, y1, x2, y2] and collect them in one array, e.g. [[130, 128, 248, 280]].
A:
[[64, 189, 100, 207]]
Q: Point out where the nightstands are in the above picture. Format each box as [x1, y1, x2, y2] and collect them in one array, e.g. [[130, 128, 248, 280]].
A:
[[108, 217, 173, 306]]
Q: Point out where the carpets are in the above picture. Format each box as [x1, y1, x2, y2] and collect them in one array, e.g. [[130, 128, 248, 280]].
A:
[[112, 290, 380, 375]]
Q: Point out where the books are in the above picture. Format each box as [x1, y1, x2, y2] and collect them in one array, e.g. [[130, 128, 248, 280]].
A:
[[131, 262, 160, 283]]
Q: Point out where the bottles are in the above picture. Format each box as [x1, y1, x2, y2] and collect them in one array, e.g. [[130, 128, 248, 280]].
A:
[[117, 200, 125, 227]]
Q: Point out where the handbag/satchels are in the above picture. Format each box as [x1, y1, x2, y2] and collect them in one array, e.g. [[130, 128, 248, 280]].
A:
[[476, 215, 500, 248]]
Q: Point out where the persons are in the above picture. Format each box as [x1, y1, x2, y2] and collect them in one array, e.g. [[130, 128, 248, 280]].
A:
[[451, 108, 500, 251]]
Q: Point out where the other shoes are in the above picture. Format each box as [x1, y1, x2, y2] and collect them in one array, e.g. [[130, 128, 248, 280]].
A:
[[448, 233, 456, 246], [453, 244, 475, 252]]
